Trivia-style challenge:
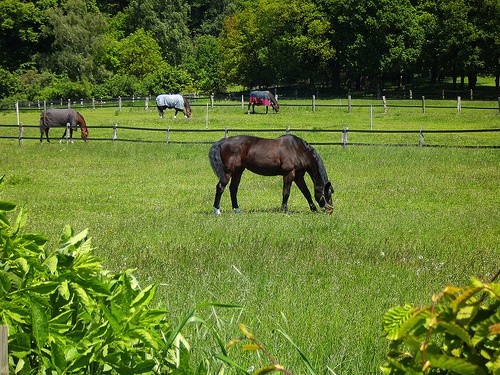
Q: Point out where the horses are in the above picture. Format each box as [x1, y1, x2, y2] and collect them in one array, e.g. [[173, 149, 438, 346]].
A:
[[247, 90, 280, 115], [39, 108, 89, 145], [208, 133, 335, 219], [155, 93, 192, 121]]
